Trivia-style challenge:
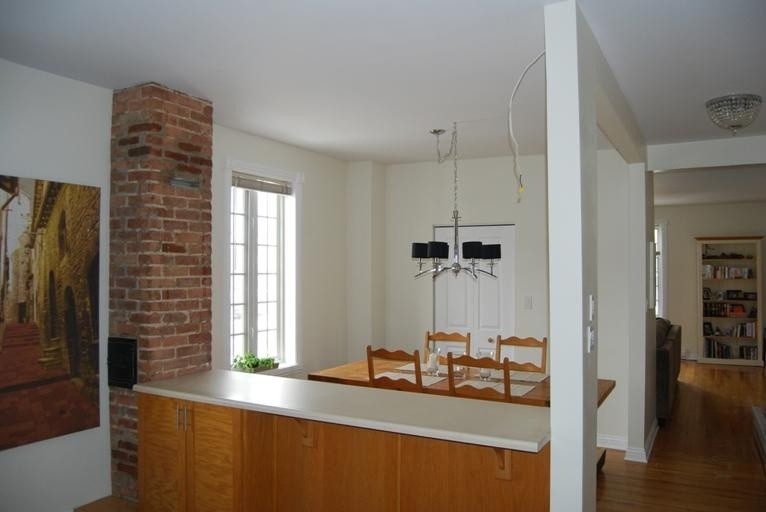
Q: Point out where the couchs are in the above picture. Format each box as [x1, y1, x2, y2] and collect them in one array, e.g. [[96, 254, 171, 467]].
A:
[[656, 318, 681, 426]]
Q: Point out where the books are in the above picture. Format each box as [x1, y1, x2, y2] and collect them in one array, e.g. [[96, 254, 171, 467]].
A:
[[701, 263, 757, 361]]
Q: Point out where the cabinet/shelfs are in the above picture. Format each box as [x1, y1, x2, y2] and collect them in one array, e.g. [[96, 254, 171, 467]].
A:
[[137, 393, 244, 512], [694, 235, 764, 366]]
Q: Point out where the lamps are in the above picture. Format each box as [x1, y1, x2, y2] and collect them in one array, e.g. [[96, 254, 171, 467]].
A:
[[410, 120, 502, 283], [704, 94, 761, 138]]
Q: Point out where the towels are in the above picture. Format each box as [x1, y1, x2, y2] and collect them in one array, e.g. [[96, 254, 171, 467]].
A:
[[475, 369, 549, 383], [454, 379, 536, 398], [394, 362, 464, 373], [374, 372, 446, 386]]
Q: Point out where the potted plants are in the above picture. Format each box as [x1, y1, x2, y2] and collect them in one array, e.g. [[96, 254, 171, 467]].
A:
[[231, 353, 280, 374]]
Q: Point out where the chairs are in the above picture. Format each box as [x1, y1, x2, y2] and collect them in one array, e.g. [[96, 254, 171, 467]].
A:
[[425, 331, 470, 357], [366, 345, 424, 393], [496, 334, 547, 371], [447, 352, 511, 402]]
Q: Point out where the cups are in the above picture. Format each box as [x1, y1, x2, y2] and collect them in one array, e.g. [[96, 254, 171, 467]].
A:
[[425, 347, 494, 382]]
